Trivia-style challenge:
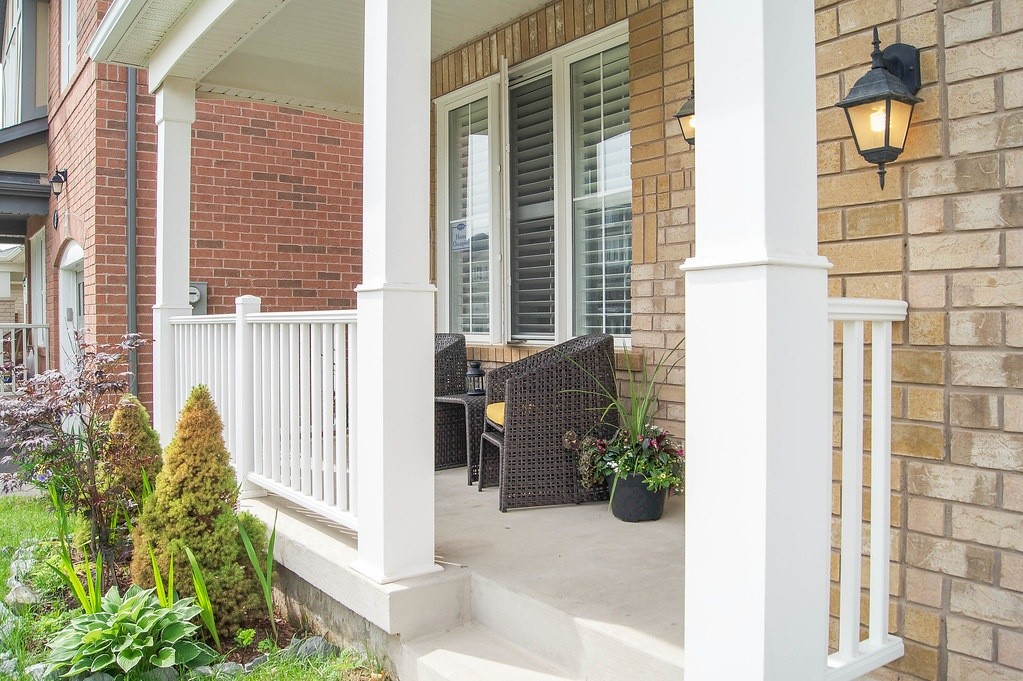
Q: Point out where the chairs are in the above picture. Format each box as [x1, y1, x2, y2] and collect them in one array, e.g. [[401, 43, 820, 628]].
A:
[[3, 328, 30, 374], [434, 333, 470, 471], [479, 331, 619, 514]]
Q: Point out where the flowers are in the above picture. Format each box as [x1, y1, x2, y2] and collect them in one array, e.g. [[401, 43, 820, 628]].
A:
[[562, 336, 685, 496]]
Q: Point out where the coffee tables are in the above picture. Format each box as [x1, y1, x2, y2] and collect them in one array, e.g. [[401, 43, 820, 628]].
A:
[[435, 395, 484, 487]]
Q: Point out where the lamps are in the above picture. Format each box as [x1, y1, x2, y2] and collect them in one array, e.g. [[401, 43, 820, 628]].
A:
[[50, 164, 70, 199], [673, 79, 694, 145], [466, 361, 485, 396], [833, 27, 920, 192]]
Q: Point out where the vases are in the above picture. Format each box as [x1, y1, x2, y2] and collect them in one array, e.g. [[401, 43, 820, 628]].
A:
[[605, 470, 667, 523]]
